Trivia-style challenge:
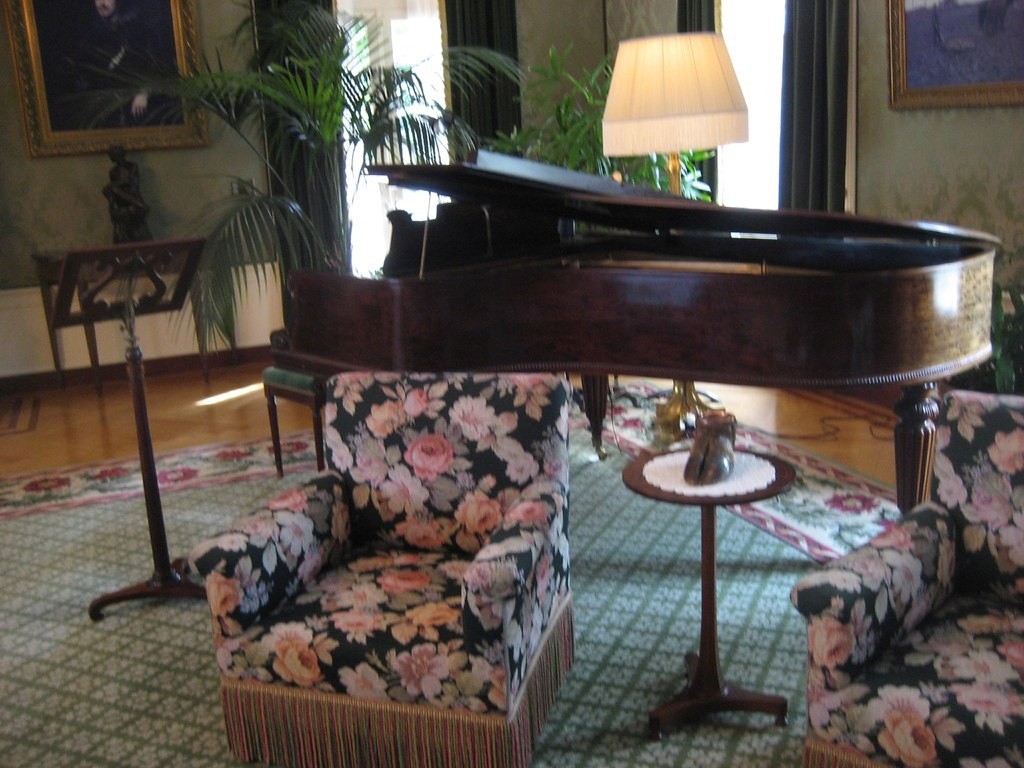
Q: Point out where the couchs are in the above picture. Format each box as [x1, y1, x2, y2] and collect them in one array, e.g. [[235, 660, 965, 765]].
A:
[[185, 369, 576, 768], [789, 390, 1024, 768]]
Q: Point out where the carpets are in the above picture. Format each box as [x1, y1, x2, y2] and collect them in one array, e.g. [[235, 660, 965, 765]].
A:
[[0, 381, 913, 768]]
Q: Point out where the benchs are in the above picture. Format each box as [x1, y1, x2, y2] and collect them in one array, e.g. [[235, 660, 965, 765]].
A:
[[263, 364, 325, 477]]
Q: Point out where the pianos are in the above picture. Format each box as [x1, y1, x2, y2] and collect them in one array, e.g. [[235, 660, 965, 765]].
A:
[[265, 150, 1003, 521]]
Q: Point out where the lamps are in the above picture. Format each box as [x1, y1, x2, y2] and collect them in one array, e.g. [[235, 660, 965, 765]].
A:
[[603, 30, 750, 443]]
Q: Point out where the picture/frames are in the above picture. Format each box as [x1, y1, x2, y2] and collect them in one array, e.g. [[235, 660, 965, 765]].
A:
[[3, 0, 209, 158], [886, 0, 1024, 112]]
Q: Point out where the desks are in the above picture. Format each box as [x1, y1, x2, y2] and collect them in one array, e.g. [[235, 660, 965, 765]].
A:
[[622, 446, 797, 741], [30, 249, 212, 396]]
